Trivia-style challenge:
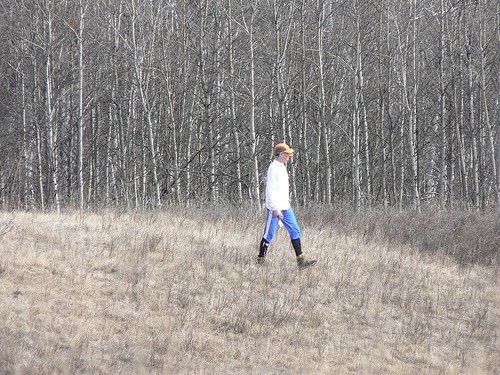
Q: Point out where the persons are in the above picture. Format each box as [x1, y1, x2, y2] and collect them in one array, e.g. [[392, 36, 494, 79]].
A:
[[257, 143, 317, 270]]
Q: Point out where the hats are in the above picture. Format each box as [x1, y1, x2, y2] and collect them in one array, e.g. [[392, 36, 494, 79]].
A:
[[274, 143, 294, 155]]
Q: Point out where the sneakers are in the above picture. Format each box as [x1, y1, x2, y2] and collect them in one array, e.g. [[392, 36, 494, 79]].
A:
[[298, 257, 317, 269]]
